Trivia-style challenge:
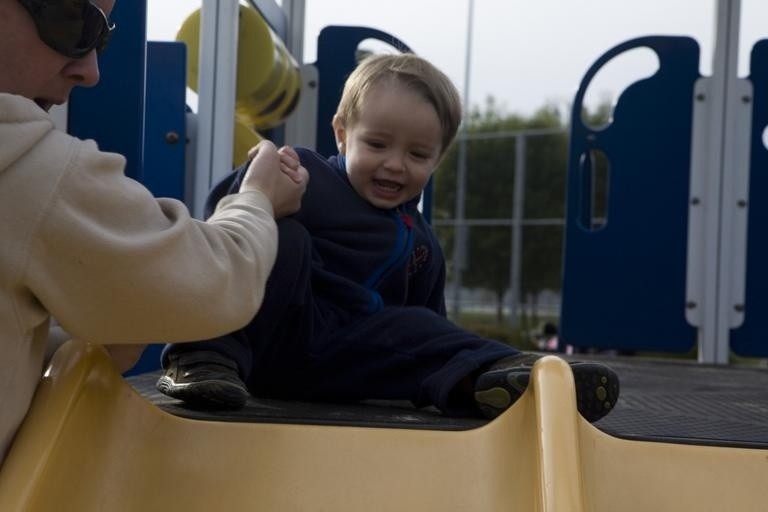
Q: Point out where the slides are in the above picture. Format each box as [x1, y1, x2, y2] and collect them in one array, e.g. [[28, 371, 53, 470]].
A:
[[1, 335, 768, 512]]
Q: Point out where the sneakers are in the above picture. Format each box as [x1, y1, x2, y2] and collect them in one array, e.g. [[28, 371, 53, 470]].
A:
[[156, 349, 249, 408], [472, 353, 620, 425]]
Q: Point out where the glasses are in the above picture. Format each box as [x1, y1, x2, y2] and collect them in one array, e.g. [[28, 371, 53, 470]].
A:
[[22, 0, 117, 59]]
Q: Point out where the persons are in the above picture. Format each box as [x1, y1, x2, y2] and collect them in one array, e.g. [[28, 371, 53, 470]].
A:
[[528, 320, 573, 355], [0, 0, 308, 463], [155, 54, 621, 422]]
